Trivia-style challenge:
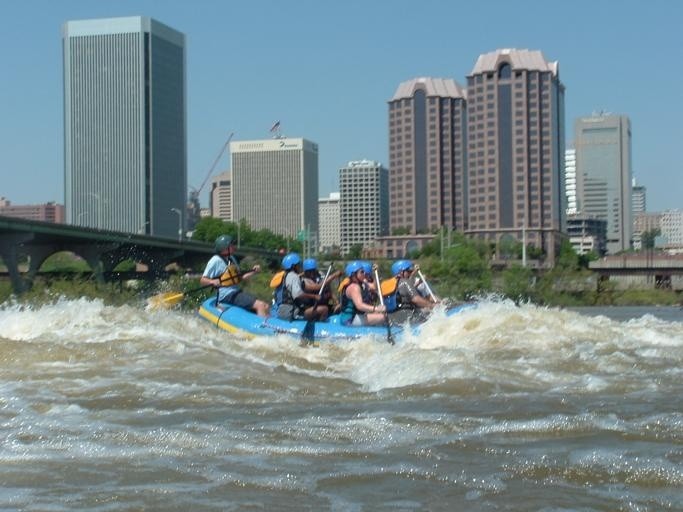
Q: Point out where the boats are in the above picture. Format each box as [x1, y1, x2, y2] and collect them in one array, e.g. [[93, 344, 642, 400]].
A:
[[196, 296, 500, 360]]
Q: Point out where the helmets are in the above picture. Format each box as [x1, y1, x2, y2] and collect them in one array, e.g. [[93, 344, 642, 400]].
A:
[[303, 258, 317, 271], [281, 252, 301, 270], [215, 234, 233, 252], [391, 259, 413, 276], [344, 260, 373, 277]]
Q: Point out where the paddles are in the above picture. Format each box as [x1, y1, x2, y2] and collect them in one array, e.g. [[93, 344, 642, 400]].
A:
[[374, 267, 394, 347], [148, 266, 254, 312], [301, 259, 334, 344]]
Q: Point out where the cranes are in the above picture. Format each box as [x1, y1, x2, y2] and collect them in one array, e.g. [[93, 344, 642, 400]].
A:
[[188, 133, 234, 197]]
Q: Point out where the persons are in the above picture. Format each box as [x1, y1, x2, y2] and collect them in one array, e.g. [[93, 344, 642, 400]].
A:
[[269, 252, 463, 329], [199, 234, 270, 317]]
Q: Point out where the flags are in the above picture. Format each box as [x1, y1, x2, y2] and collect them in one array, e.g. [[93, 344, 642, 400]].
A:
[[271, 121, 281, 132]]
